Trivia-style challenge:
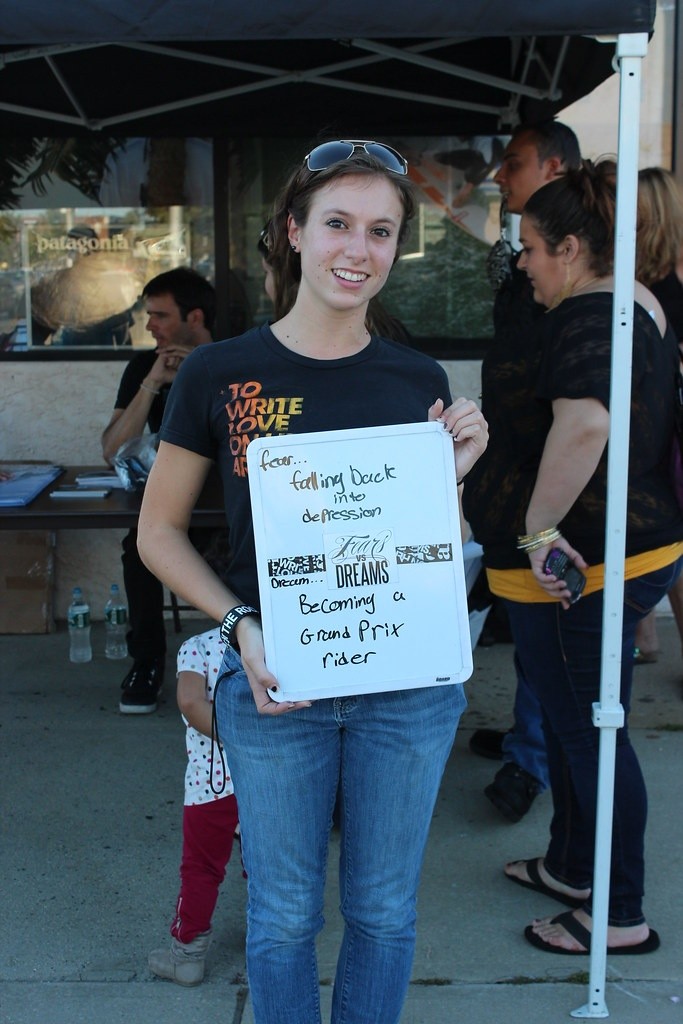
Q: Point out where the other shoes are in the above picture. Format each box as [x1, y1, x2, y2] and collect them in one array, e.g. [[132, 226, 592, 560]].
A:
[[486, 760, 538, 821], [469, 729, 505, 760]]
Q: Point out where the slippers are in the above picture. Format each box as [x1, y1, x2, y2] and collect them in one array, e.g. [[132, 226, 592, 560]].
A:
[[525, 910, 661, 954], [632, 647, 660, 662], [505, 856, 590, 909]]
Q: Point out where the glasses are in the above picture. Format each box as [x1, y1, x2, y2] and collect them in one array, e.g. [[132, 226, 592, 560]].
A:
[[302, 139, 409, 175]]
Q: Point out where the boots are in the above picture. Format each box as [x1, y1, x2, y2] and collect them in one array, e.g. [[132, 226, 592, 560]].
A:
[[148, 928, 212, 985]]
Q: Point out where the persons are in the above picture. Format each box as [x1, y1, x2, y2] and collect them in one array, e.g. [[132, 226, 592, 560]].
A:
[[142, 532, 246, 988], [480, 155, 683, 958], [627, 168, 683, 691], [133, 138, 490, 1024], [102, 265, 232, 713], [467, 121, 582, 824], [257, 210, 416, 352], [29, 222, 134, 346]]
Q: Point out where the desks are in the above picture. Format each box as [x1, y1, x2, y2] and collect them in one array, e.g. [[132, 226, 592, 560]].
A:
[[0, 465, 228, 528]]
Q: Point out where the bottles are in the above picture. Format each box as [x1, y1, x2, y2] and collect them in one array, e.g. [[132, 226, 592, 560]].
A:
[[103, 585, 128, 660], [67, 588, 93, 663]]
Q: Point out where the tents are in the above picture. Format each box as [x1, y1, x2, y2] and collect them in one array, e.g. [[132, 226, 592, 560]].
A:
[[0, 0, 658, 1017]]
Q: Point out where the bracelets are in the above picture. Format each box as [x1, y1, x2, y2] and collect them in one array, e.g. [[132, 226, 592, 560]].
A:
[[457, 479, 463, 486], [516, 527, 561, 553], [219, 604, 261, 657], [140, 384, 159, 394]]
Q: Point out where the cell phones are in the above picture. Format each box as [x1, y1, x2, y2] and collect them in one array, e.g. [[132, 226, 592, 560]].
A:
[[544, 548, 587, 605]]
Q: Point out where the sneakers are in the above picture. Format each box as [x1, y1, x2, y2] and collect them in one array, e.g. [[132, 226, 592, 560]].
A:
[[119, 663, 162, 713]]
